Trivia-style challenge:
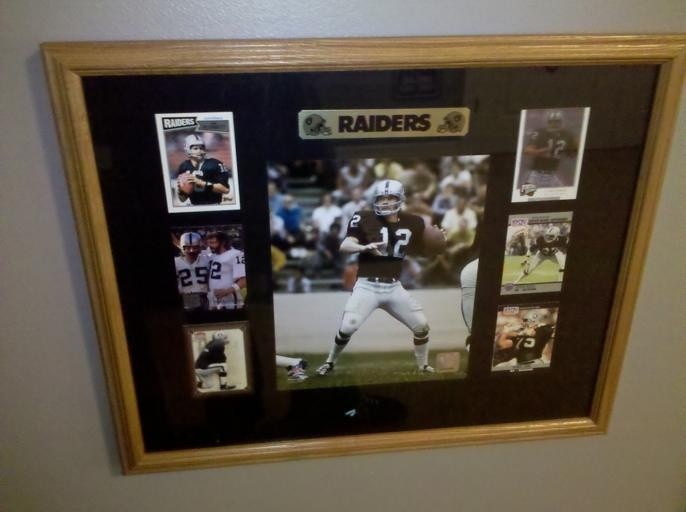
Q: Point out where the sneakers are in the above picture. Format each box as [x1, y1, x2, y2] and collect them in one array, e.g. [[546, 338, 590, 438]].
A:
[[220, 384, 235, 389], [288, 359, 306, 377], [419, 365, 435, 373], [317, 362, 334, 376]]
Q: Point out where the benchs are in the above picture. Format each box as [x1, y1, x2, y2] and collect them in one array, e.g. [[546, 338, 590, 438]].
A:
[[273, 176, 349, 291]]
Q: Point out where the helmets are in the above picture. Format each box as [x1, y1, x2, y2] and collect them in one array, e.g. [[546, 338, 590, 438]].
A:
[[545, 227, 560, 242], [181, 232, 202, 254], [214, 332, 229, 344], [524, 309, 550, 326], [547, 113, 565, 131], [184, 134, 206, 160], [373, 179, 404, 216]]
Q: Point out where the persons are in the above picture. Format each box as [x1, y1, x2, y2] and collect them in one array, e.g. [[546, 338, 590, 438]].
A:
[[497, 308, 554, 372], [269, 157, 489, 386], [527, 112, 576, 189], [194, 331, 236, 390], [176, 132, 231, 207], [174, 229, 245, 311], [508, 220, 571, 287]]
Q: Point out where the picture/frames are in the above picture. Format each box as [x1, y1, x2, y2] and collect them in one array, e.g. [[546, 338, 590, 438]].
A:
[[38, 30, 686, 475]]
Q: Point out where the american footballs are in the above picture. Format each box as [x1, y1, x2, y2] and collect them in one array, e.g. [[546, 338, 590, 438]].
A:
[[178, 173, 193, 195], [424, 226, 445, 253]]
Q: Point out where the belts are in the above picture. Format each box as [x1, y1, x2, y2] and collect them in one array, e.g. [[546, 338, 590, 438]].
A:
[[368, 277, 397, 282]]
